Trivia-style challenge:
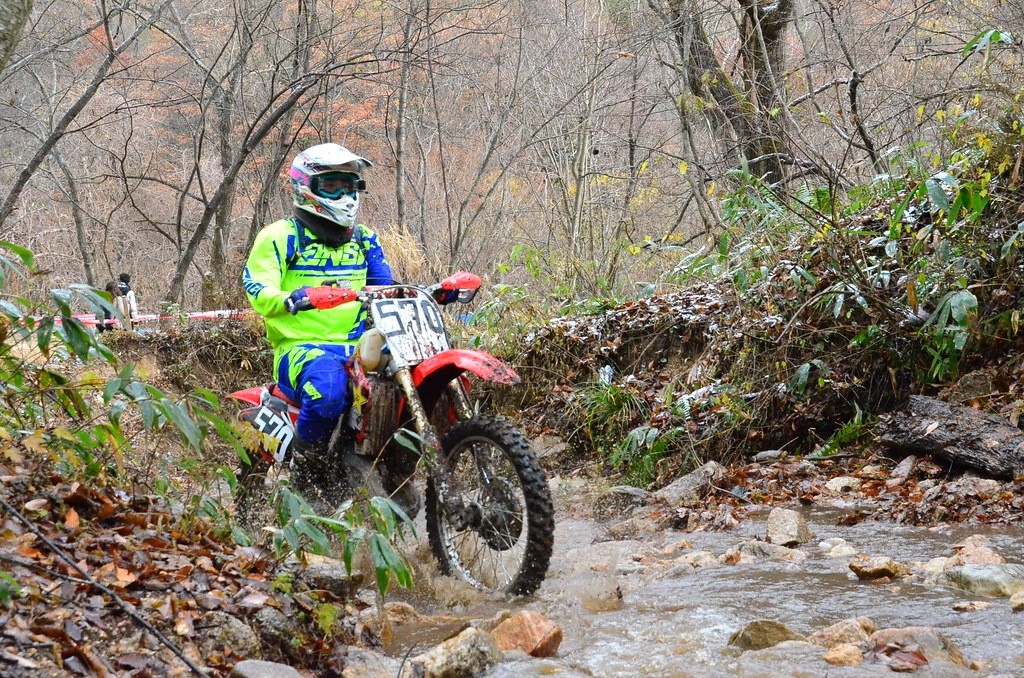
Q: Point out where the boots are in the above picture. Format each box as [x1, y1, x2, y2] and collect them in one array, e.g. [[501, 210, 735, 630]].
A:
[[287, 431, 345, 535]]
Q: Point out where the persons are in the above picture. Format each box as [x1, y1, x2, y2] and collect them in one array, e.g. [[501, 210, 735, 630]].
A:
[[242, 145, 395, 519], [95, 282, 124, 332], [118, 273, 139, 332]]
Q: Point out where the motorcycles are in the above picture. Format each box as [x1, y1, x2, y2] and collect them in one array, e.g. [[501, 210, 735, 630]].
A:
[[226, 270, 557, 607]]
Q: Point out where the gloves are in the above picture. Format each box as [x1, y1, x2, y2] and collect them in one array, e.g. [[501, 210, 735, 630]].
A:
[[434, 288, 460, 305], [284, 286, 316, 315]]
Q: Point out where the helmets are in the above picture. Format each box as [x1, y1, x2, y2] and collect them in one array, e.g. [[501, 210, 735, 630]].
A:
[[289, 143, 374, 248]]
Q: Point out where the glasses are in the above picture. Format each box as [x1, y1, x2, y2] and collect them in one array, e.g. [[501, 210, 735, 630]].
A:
[[310, 173, 357, 201]]
